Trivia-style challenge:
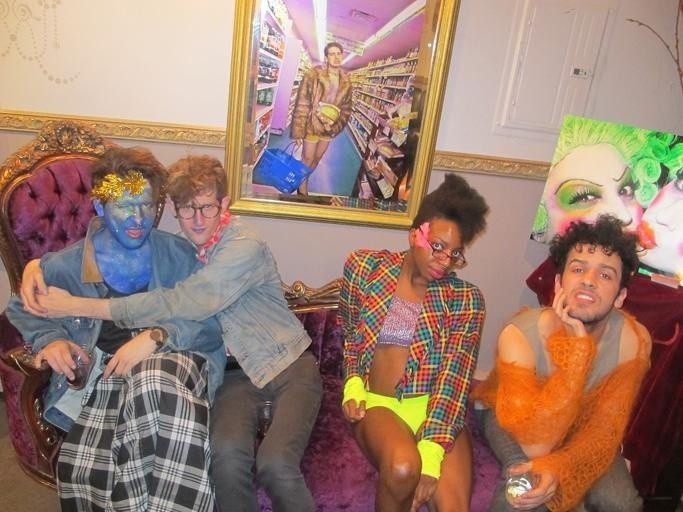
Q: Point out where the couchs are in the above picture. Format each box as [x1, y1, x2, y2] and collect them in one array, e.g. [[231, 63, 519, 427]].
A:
[[1, 122, 504, 511]]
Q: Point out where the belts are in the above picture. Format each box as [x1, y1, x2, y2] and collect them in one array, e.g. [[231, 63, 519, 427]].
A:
[[220, 355, 244, 373]]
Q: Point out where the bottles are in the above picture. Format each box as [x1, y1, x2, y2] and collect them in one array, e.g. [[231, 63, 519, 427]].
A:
[[255, 22, 285, 107]]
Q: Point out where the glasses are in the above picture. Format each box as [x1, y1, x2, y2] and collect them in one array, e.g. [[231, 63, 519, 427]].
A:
[[173, 200, 222, 220], [416, 225, 466, 267]]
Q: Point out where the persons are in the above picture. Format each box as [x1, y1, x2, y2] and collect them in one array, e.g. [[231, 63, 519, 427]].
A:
[[530, 115, 683, 277], [5, 144, 227, 512], [334, 174, 491, 512], [289, 42, 351, 195], [19, 154, 324, 512], [483, 213, 653, 511]]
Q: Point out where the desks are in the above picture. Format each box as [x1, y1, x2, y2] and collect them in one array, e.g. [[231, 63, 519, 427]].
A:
[[530, 252, 681, 498]]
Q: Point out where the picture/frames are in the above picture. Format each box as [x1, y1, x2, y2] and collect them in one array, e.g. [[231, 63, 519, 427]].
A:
[[220, 0, 464, 230]]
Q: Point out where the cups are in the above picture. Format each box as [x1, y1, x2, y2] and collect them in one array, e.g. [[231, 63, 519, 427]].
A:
[[503, 460, 538, 505], [64, 344, 97, 391], [254, 400, 274, 435]]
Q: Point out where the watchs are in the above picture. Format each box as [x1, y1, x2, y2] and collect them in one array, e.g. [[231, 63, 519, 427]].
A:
[[149, 328, 163, 346]]
